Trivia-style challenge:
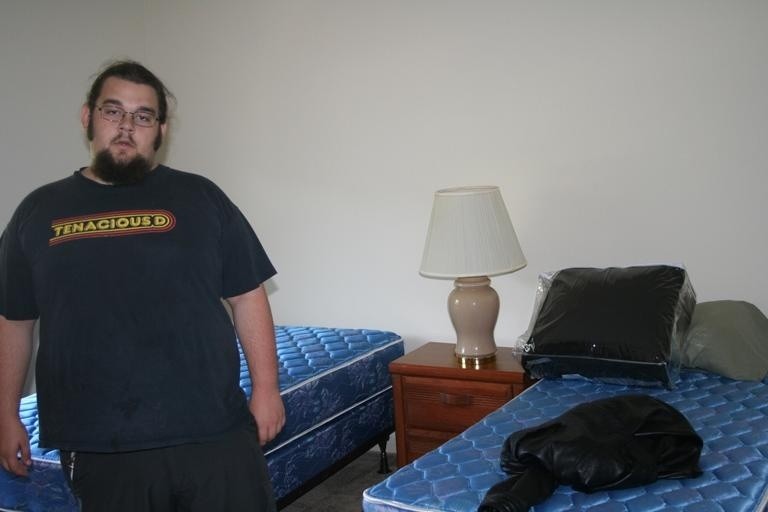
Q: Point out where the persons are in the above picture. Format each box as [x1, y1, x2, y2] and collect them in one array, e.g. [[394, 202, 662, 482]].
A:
[[2, 60, 294, 510]]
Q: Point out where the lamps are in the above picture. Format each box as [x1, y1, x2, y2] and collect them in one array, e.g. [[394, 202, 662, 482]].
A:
[[419, 186, 527, 361]]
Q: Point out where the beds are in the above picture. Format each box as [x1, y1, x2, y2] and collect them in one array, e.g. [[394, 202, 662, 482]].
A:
[[361, 364, 767, 510], [1, 326, 403, 511]]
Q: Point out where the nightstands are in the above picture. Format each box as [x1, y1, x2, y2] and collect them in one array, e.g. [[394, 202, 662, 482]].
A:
[[389, 341, 527, 469]]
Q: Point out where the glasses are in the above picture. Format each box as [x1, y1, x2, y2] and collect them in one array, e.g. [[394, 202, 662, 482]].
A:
[[94, 103, 161, 128]]
